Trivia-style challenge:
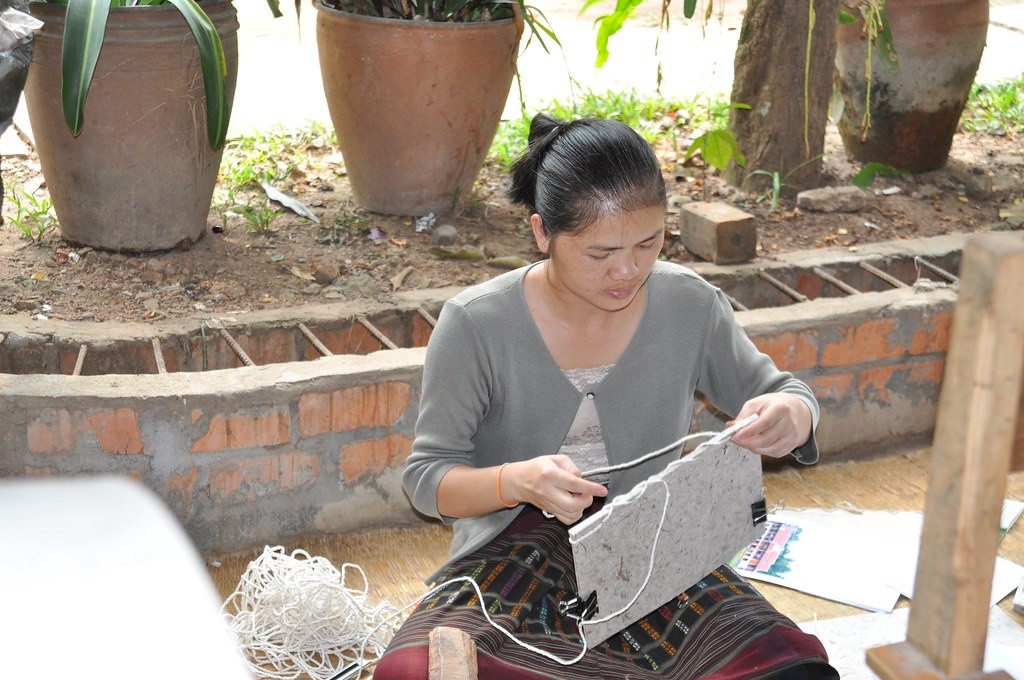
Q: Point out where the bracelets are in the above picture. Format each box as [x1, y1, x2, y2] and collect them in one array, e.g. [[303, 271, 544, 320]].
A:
[[497, 463, 520, 508]]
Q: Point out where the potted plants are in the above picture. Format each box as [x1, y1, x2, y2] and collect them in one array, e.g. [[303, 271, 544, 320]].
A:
[[833, 0, 990, 171], [311, 0, 562, 216], [23, 0, 240, 252]]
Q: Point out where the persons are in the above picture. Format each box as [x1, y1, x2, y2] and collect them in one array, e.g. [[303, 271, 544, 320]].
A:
[[371, 114, 840, 680]]
